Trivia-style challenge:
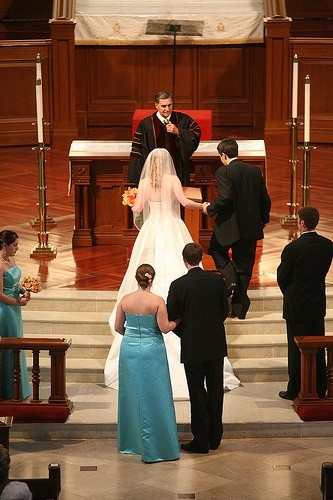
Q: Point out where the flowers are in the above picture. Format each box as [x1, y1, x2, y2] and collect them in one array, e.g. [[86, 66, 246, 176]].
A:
[[121, 186, 138, 208], [19, 276, 42, 300]]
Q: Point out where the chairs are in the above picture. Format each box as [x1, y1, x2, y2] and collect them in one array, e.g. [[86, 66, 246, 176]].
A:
[[131, 139, 135, 140]]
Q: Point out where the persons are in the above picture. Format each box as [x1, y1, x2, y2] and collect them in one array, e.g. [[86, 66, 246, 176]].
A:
[[0, 444, 32, 500], [277, 207, 333, 400], [115, 264, 183, 463], [167, 243, 229, 453], [129, 91, 202, 186], [104, 148, 240, 400], [0, 230, 31, 400], [202, 138, 272, 319]]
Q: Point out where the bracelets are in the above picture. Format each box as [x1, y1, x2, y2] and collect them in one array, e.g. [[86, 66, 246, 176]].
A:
[[176, 129, 179, 138]]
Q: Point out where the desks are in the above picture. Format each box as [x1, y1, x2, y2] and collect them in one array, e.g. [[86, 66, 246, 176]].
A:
[[68, 140, 266, 249]]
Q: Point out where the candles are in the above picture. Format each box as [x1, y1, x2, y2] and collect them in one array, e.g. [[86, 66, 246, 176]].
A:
[[293, 53, 298, 118], [35, 53, 44, 143], [304, 74, 311, 142]]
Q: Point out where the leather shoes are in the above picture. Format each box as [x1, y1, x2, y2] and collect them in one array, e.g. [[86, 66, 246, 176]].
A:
[[181, 443, 209, 453], [225, 282, 237, 298], [279, 390, 296, 400], [226, 312, 245, 319]]
